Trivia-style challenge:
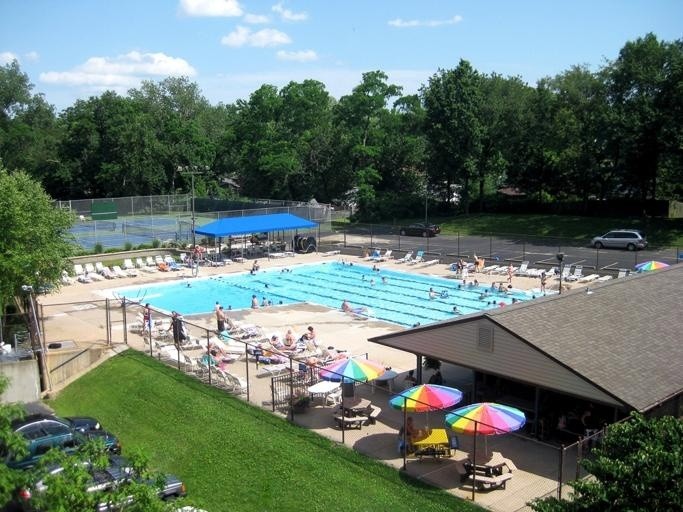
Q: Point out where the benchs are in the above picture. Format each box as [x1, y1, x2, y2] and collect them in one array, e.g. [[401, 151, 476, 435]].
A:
[[326, 388, 517, 490]]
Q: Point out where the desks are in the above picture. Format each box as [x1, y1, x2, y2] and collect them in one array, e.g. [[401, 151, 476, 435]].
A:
[[308, 381, 340, 404]]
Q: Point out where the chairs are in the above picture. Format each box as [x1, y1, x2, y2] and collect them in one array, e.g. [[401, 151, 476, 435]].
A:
[[447, 258, 628, 280], [364, 250, 380, 261], [375, 251, 394, 262], [398, 251, 413, 264], [322, 251, 340, 257], [61, 243, 293, 284], [131, 312, 348, 395], [408, 252, 424, 265]]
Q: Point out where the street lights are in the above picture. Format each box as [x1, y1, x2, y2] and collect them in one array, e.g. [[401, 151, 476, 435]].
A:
[[21, 285, 52, 390], [176, 164, 211, 247], [556, 252, 564, 294]]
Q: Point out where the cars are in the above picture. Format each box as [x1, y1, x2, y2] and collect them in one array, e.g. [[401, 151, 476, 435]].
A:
[[398, 222, 440, 237], [0, 400, 207, 512], [591, 229, 649, 251]]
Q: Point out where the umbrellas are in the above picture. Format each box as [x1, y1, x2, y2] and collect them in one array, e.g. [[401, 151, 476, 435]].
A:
[[634, 260, 669, 272], [444, 402, 527, 457], [388, 382, 464, 437]]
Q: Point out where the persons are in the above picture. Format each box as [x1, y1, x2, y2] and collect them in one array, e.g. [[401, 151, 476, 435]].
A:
[[429, 371, 443, 385], [159, 245, 202, 272], [399, 417, 419, 453], [405, 370, 416, 390], [580, 411, 592, 434], [142, 293, 346, 413], [186, 284, 191, 288], [223, 236, 260, 259], [250, 250, 569, 328]]
